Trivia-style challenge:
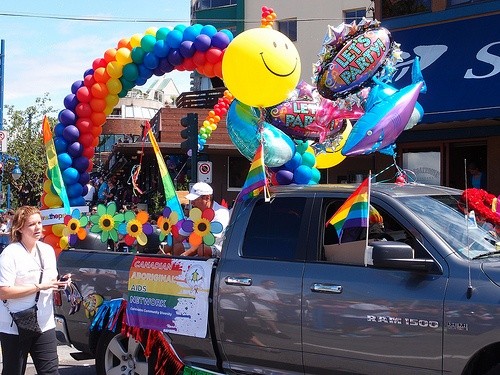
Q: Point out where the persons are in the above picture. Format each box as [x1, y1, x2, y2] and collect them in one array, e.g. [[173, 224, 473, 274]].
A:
[[467, 161, 485, 189], [0, 168, 232, 260], [0, 205, 72, 375], [447, 207, 494, 247]]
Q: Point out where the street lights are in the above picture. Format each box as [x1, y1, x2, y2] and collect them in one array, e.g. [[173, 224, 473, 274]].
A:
[[3, 152, 22, 210]]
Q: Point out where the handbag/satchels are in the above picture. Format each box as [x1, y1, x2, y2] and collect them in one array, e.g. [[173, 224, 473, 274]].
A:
[[10, 305, 43, 343]]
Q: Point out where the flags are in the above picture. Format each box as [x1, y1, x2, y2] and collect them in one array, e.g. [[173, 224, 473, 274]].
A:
[[237, 144, 270, 202], [325, 171, 372, 246]]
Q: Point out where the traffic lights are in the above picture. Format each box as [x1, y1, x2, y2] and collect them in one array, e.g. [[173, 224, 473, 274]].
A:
[[181, 112, 199, 152]]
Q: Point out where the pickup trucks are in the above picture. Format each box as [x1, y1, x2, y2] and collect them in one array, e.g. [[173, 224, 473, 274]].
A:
[[53, 180, 500, 375]]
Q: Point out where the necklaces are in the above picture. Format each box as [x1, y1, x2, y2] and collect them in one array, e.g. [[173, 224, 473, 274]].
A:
[[23, 242, 44, 272]]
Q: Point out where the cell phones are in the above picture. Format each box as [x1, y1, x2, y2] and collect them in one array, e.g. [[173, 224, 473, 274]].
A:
[[59, 278, 68, 289]]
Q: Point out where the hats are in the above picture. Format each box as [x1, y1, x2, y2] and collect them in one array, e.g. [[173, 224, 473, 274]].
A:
[[184, 182, 213, 200]]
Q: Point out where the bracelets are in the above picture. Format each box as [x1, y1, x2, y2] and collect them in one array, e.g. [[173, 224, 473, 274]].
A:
[[33, 283, 40, 290]]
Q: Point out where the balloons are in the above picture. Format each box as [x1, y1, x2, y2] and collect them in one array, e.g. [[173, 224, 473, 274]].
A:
[[37, 6, 427, 250]]
